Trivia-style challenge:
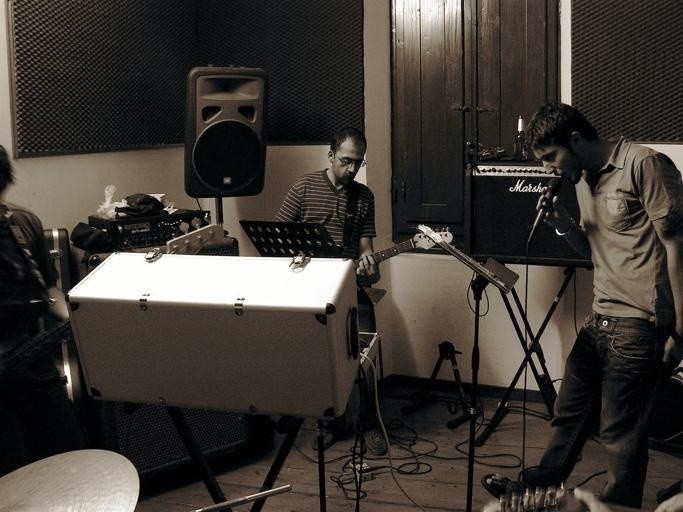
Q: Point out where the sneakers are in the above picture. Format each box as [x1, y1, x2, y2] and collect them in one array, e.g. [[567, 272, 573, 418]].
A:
[[357, 419, 388, 455], [312, 420, 350, 450], [479, 471, 525, 503]]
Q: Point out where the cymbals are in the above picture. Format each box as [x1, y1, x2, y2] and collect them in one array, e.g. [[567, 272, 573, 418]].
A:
[[1, 449, 140, 512]]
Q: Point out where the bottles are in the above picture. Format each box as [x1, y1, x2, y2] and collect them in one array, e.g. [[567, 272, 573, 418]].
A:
[[513, 113, 530, 162]]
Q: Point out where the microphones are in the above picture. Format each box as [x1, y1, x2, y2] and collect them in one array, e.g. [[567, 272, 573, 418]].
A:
[[527, 177, 561, 244]]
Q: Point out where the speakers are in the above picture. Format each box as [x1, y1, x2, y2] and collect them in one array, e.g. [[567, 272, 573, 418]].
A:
[[183, 66, 270, 197], [648, 378, 681, 458], [464, 162, 593, 269]]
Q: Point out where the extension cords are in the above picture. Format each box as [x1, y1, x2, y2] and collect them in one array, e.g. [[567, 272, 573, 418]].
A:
[[351, 460, 372, 483]]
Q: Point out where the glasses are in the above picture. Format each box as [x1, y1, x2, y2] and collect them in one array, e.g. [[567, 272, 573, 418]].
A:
[[333, 152, 366, 169]]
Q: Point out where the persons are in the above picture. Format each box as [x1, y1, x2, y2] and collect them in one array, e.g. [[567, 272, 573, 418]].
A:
[[1, 147, 73, 475], [481, 102, 683, 512], [275, 125, 389, 456]]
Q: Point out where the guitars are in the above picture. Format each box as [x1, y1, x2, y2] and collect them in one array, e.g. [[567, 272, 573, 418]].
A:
[[355, 227, 453, 271], [0, 223, 224, 378]]
